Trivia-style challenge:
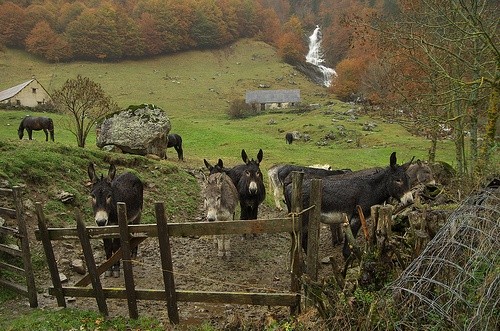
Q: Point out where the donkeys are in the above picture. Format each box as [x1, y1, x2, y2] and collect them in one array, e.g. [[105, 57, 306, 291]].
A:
[[237, 148, 266, 240], [200, 172, 239, 257], [285, 152, 416, 263], [17, 116, 54, 142], [268, 161, 332, 211], [167, 134, 183, 160], [277, 164, 353, 205], [204, 158, 244, 187], [285, 133, 293, 145], [322, 158, 435, 246], [88, 164, 144, 278]]
